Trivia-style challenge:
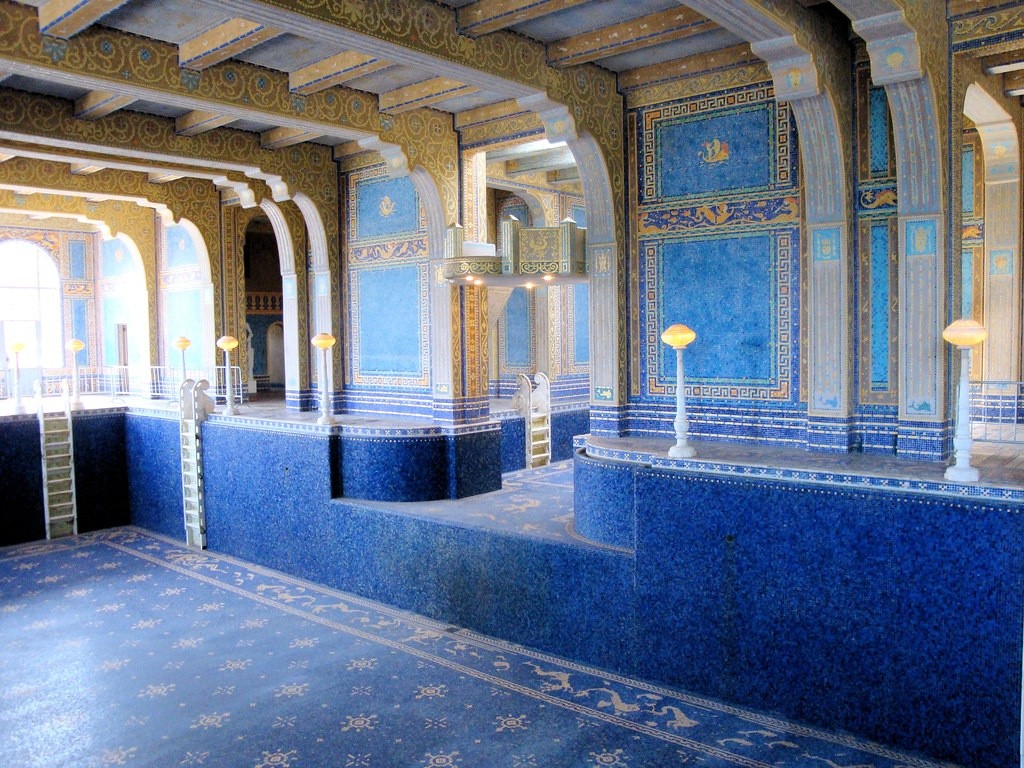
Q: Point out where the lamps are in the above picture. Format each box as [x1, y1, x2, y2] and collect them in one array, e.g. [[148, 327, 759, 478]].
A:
[[942, 319, 987, 346], [173, 337, 190, 349], [661, 323, 696, 347], [67, 339, 84, 353], [217, 335, 239, 352], [311, 333, 335, 350]]
